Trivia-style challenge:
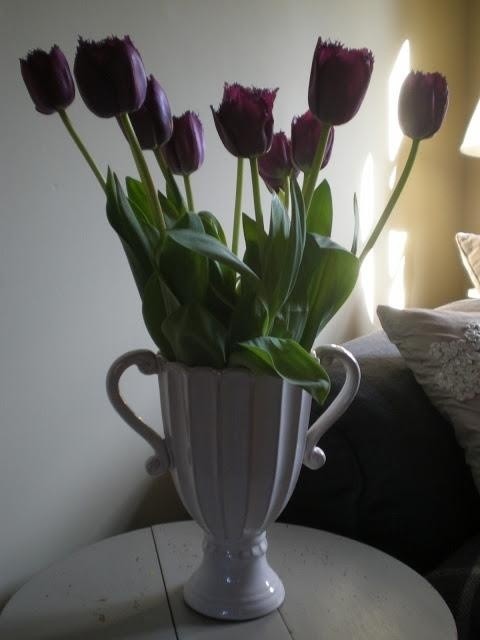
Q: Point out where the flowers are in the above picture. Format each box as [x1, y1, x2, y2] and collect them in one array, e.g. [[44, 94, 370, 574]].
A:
[[18, 33, 448, 404]]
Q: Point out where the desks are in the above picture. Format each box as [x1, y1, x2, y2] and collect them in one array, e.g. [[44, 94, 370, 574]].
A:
[[0, 520, 458, 639]]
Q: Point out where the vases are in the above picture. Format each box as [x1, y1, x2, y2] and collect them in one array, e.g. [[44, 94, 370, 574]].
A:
[[106, 343, 362, 620]]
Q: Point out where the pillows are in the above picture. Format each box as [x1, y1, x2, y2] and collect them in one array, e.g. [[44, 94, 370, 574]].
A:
[[376, 305, 480, 489], [455, 232, 480, 293]]
